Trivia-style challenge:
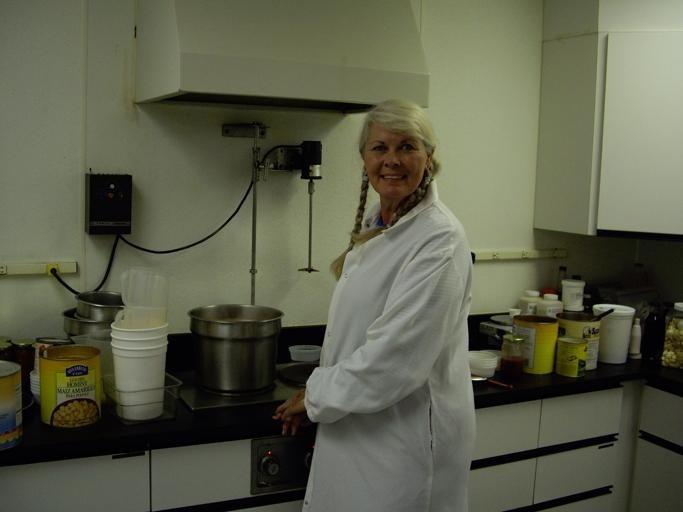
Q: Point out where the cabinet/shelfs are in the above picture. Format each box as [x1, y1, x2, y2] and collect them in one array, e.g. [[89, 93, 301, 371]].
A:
[[533, 1, 683, 237]]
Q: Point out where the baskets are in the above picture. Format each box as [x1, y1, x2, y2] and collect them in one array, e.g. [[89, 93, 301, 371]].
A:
[[101, 371, 183, 424]]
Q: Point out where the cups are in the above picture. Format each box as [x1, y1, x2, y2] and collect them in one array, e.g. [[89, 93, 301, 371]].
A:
[[109, 319, 169, 422]]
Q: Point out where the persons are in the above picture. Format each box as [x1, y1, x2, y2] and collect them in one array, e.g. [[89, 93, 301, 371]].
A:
[[269, 94, 478, 512]]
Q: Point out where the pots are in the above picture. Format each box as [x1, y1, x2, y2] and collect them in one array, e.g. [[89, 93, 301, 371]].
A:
[[187, 304, 286, 391], [61, 290, 126, 344]]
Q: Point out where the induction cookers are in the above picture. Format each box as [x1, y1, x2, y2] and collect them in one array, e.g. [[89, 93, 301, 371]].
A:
[[171, 362, 319, 412]]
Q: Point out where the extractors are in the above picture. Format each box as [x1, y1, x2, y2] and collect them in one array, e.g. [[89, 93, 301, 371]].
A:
[[132, 0, 430, 115]]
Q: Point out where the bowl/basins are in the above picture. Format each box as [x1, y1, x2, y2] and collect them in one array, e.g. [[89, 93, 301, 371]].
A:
[[468, 350, 502, 378], [288, 344, 322, 362]]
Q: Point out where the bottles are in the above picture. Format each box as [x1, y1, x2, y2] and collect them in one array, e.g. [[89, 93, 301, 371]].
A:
[[518, 265, 586, 319], [628, 318, 642, 355], [661, 302, 683, 369], [500, 333, 525, 379], [0, 335, 36, 409]]
